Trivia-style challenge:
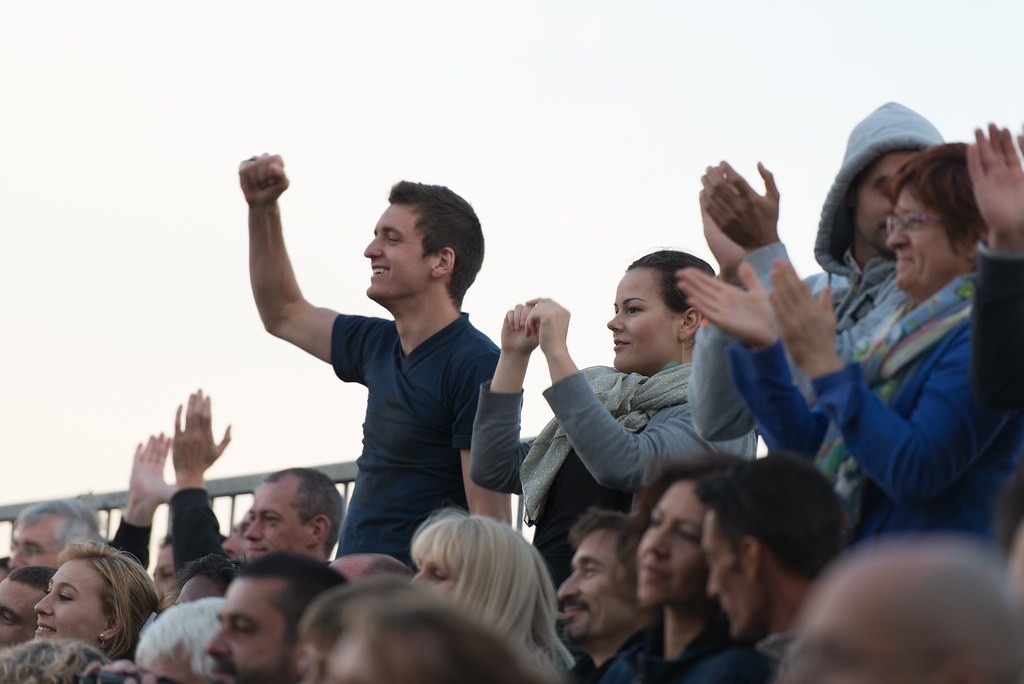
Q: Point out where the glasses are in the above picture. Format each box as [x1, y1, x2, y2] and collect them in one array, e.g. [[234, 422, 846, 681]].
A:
[[886, 212, 943, 233]]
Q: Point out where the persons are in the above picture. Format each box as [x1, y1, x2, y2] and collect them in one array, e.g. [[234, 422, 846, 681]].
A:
[[592, 452, 772, 684], [468, 252, 755, 590], [408, 510, 577, 684], [237, 152, 514, 574], [291, 551, 548, 684], [693, 453, 842, 684], [774, 529, 1024, 684], [688, 101, 947, 440], [966, 122, 1024, 407], [0, 388, 354, 684], [552, 508, 654, 684], [676, 141, 1024, 559]]
